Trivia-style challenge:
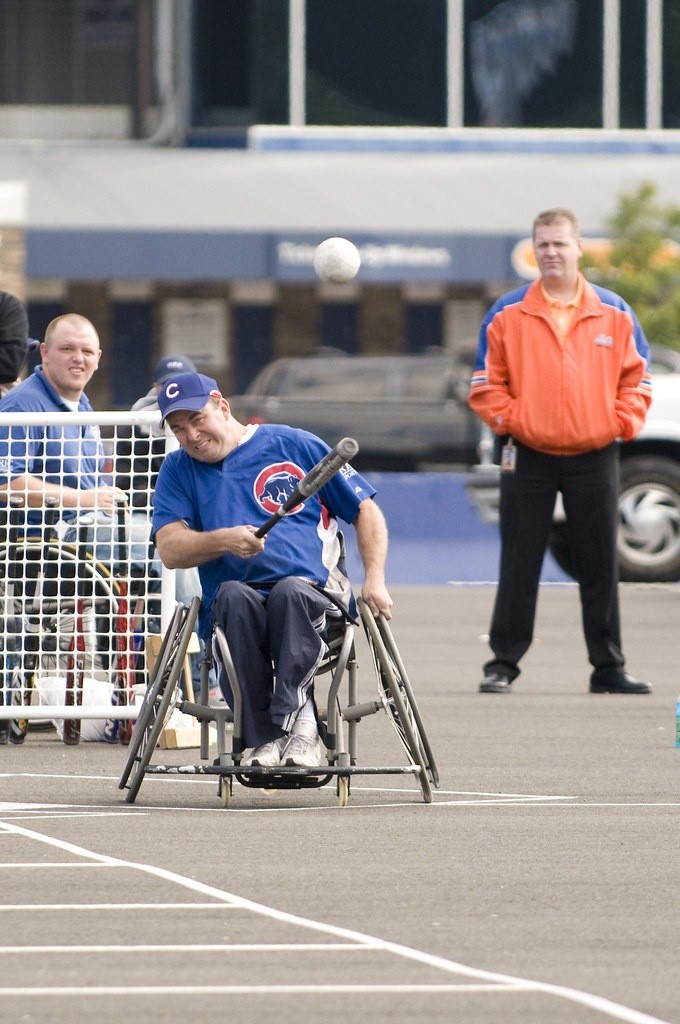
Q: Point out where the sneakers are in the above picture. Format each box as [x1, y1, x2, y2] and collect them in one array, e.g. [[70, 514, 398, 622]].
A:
[[280, 734, 325, 766], [246, 735, 289, 768]]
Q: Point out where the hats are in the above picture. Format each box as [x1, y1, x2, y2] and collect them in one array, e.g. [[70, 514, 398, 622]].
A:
[[152, 354, 197, 382], [157, 372, 220, 430]]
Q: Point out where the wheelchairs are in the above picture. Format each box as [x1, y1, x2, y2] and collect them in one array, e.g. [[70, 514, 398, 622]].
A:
[[116, 583, 444, 814], [0, 493, 227, 733]]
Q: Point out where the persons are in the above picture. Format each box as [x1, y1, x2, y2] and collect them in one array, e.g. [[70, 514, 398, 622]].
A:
[[153, 371, 391, 784], [0, 314, 218, 701], [0, 289, 29, 384], [114, 355, 197, 516], [466, 209, 653, 694]]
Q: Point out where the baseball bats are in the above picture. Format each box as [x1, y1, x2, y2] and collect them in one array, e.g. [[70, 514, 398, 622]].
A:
[[0, 493, 155, 748], [253, 435, 360, 539]]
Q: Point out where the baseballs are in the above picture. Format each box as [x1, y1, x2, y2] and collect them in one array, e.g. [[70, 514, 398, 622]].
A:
[[313, 236, 361, 285]]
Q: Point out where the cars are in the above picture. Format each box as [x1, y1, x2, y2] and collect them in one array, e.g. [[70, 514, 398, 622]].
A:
[[467, 348, 679, 585]]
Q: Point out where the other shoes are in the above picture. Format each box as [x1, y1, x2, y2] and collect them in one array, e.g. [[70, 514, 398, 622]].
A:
[[479, 671, 510, 693], [589, 672, 652, 693]]
